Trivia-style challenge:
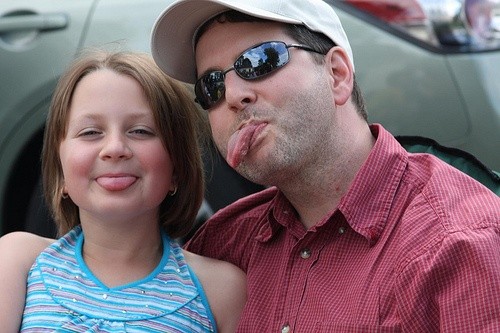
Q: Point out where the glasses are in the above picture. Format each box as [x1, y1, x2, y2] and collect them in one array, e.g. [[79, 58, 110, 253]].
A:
[[194, 42, 327, 111]]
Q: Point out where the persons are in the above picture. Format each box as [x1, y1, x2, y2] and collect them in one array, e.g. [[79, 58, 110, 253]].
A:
[[0, 52, 247, 333], [151, 0, 500, 333]]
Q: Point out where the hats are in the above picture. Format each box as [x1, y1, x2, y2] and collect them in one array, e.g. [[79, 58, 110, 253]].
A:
[[150, 0, 354, 84]]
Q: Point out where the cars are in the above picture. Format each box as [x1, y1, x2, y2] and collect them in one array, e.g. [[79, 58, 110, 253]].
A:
[[0, 0, 500, 247]]
